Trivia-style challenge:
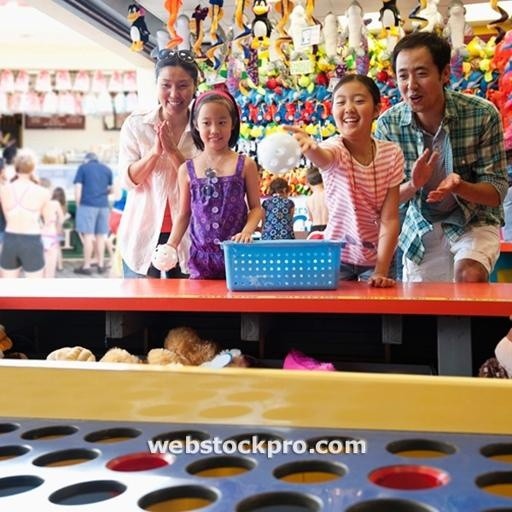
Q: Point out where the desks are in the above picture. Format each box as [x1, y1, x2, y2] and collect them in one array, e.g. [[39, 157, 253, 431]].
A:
[[0, 277, 512, 378]]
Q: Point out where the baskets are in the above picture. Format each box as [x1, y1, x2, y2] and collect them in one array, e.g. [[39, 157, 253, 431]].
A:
[[223, 241, 343, 291]]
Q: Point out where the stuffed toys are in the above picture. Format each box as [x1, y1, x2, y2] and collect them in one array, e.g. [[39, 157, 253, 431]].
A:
[[126, 1, 511, 196], [1, 316, 247, 368], [127, 0, 511, 196]]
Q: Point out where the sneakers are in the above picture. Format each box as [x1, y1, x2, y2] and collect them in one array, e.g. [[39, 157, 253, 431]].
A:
[[74, 267, 107, 276]]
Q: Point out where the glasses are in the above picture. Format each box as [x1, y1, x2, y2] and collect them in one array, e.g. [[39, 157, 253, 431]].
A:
[[158, 49, 195, 63], [203, 167, 217, 196]]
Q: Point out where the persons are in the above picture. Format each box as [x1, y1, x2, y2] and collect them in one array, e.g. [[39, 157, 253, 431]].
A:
[[0, 154, 67, 279], [373, 33, 509, 284], [121, 50, 202, 279], [283, 75, 404, 288], [163, 91, 264, 282], [73, 152, 113, 275], [307, 172, 329, 233], [262, 177, 294, 239]]
